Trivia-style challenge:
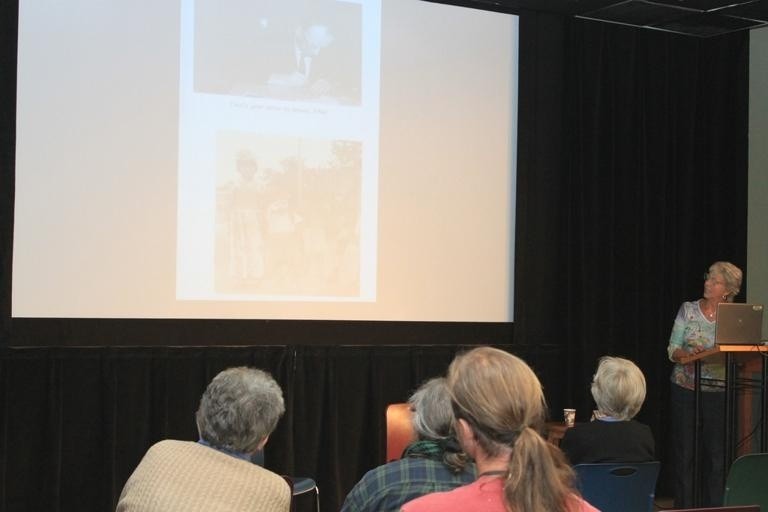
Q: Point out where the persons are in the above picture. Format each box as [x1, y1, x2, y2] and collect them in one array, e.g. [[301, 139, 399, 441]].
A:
[[664, 260, 742, 509], [559, 356, 658, 476], [337, 374, 479, 511], [111, 364, 292, 512], [397, 345, 602, 511]]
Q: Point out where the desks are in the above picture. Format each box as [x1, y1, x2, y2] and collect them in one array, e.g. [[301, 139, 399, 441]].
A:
[[542, 420, 577, 446]]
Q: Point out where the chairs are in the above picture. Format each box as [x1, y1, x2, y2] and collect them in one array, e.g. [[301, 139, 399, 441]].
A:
[[386, 403, 417, 461], [281, 473, 319, 512], [571, 461, 660, 511], [723, 453, 768, 512]]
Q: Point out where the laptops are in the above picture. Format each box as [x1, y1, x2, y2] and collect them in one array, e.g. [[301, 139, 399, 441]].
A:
[[694, 303, 764, 356]]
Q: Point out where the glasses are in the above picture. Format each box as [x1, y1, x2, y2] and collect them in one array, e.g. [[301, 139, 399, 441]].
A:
[[704, 273, 729, 286]]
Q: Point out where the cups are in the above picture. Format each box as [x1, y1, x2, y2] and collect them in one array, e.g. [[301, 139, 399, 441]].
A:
[[564, 409, 576, 429]]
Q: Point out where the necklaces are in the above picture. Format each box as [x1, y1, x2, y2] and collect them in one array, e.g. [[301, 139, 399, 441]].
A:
[[701, 301, 715, 319]]
